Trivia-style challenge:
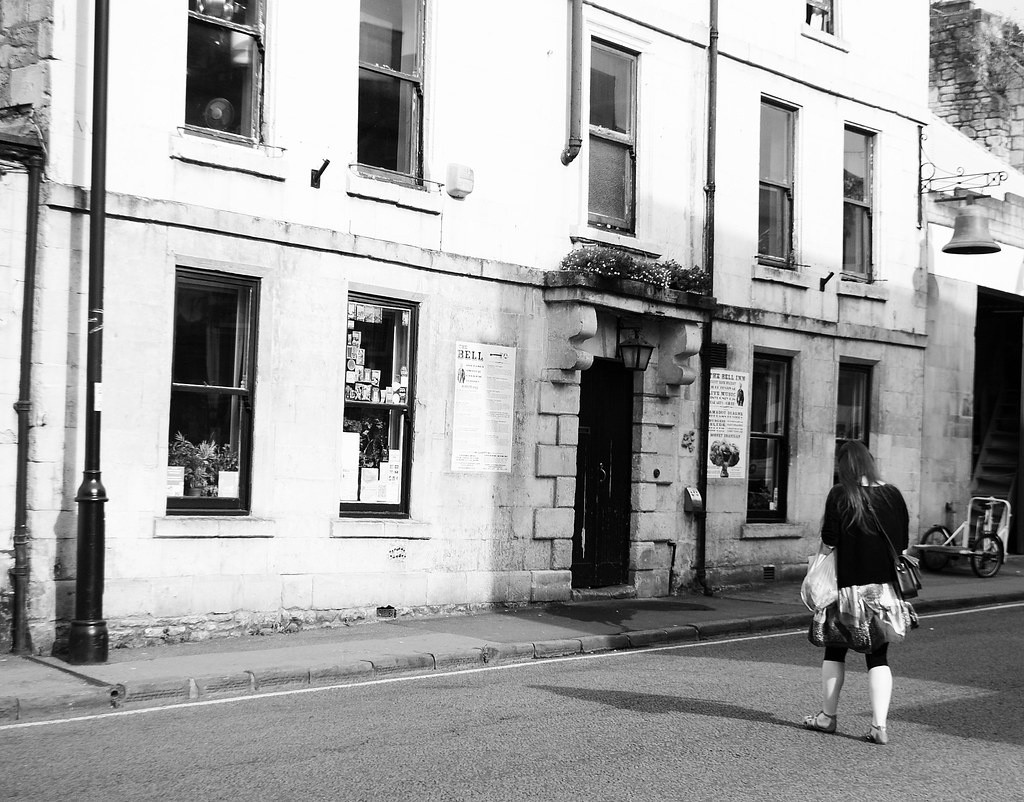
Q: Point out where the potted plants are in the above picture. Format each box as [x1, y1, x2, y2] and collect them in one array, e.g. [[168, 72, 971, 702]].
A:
[[167, 431, 237, 497]]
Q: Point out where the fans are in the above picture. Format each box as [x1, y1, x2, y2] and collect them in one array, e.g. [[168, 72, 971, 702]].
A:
[[204, 98, 235, 132]]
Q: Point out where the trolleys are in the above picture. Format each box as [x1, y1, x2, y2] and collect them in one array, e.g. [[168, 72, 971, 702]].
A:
[[912, 496, 1012, 579]]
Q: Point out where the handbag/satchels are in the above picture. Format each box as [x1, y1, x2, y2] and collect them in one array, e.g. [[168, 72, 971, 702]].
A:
[[800, 542, 839, 613], [894, 554, 922, 600]]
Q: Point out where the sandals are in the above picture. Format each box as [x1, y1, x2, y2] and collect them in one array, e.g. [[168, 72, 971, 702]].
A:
[[865, 724, 888, 744], [804, 711, 837, 734]]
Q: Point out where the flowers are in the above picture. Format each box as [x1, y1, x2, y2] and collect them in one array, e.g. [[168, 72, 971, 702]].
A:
[[561, 246, 711, 297]]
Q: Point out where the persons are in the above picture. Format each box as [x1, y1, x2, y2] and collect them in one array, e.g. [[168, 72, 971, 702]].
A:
[[802, 440, 910, 745]]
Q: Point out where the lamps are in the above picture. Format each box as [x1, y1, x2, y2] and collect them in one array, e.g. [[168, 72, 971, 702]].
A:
[[614, 314, 656, 370], [935, 194, 1001, 255]]
